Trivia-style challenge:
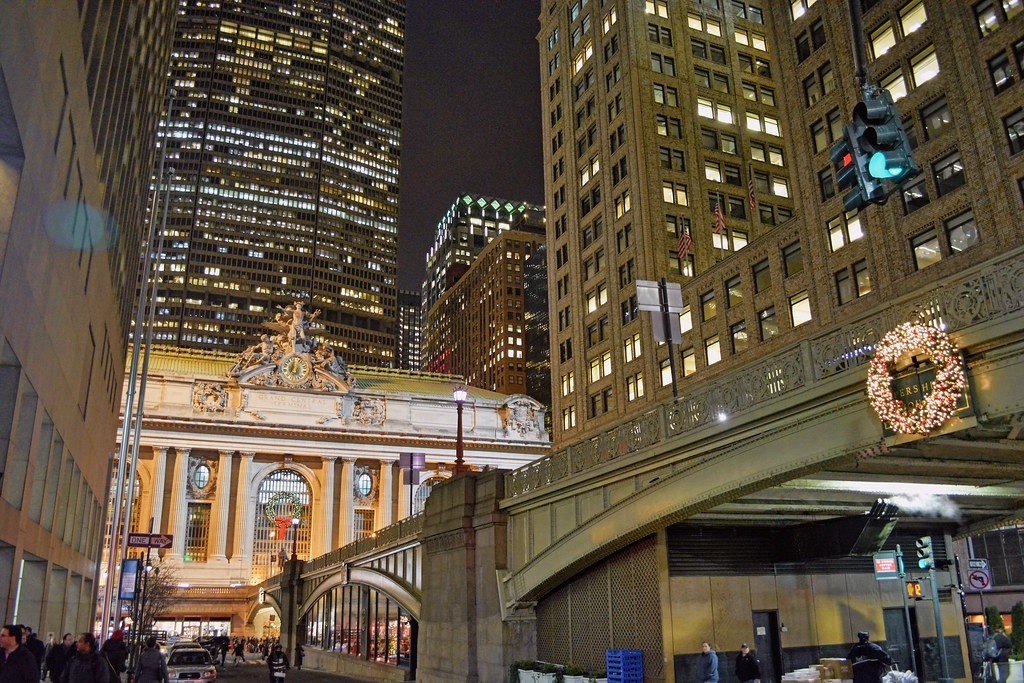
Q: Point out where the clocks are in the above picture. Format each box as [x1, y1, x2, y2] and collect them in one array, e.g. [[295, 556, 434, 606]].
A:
[[279, 353, 311, 385]]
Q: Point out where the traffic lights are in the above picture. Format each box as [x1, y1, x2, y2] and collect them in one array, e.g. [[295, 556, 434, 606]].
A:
[[828, 121, 890, 213], [853, 88, 918, 186], [914, 536, 935, 571]]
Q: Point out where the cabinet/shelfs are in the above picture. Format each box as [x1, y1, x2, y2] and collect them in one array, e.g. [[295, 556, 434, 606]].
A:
[[607, 649, 643, 683]]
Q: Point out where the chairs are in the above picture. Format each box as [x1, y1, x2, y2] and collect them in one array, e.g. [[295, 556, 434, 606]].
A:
[[177, 656, 184, 662], [195, 656, 204, 662], [186, 657, 193, 662]]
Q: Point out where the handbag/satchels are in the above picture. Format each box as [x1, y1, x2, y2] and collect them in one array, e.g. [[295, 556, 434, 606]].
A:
[[119, 672, 129, 683], [300, 651, 305, 656]]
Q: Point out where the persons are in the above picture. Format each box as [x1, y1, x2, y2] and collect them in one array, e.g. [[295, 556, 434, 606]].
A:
[[250, 334, 277, 366], [202, 630, 280, 665], [696, 641, 719, 683], [315, 341, 344, 373], [734, 643, 761, 683], [277, 301, 322, 353], [983, 625, 1012, 683], [294, 643, 305, 672], [266, 644, 291, 683], [0, 624, 170, 683], [334, 635, 410, 664], [845, 632, 895, 683]]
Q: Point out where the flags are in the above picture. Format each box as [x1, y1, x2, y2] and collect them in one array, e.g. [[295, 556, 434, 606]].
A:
[[747, 166, 757, 212], [715, 198, 726, 234], [678, 223, 692, 259]]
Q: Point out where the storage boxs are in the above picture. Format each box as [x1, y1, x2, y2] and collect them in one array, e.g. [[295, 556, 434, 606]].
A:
[[821, 658, 853, 679]]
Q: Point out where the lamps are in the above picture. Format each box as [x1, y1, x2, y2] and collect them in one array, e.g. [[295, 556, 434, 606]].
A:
[[452, 386, 471, 476], [291, 517, 299, 560]]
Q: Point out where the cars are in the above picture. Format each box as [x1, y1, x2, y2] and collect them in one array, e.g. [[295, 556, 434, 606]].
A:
[[162, 648, 221, 683], [168, 635, 219, 659]]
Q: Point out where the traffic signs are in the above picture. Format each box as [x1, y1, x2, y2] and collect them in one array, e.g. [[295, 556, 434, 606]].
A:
[[968, 558, 988, 570], [127, 531, 174, 549]]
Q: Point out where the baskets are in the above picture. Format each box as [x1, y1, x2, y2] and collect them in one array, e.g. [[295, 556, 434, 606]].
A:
[[605, 650, 643, 683]]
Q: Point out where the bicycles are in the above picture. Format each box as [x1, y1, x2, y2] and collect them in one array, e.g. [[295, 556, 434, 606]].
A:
[[270, 666, 290, 683]]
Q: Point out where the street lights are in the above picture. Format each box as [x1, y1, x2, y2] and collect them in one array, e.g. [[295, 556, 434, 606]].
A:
[[451, 386, 471, 476], [291, 516, 300, 561], [128, 551, 153, 655]]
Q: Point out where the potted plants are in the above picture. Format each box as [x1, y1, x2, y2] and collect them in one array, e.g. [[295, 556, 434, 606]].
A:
[[982, 651, 1024, 683], [509, 659, 609, 683]]
